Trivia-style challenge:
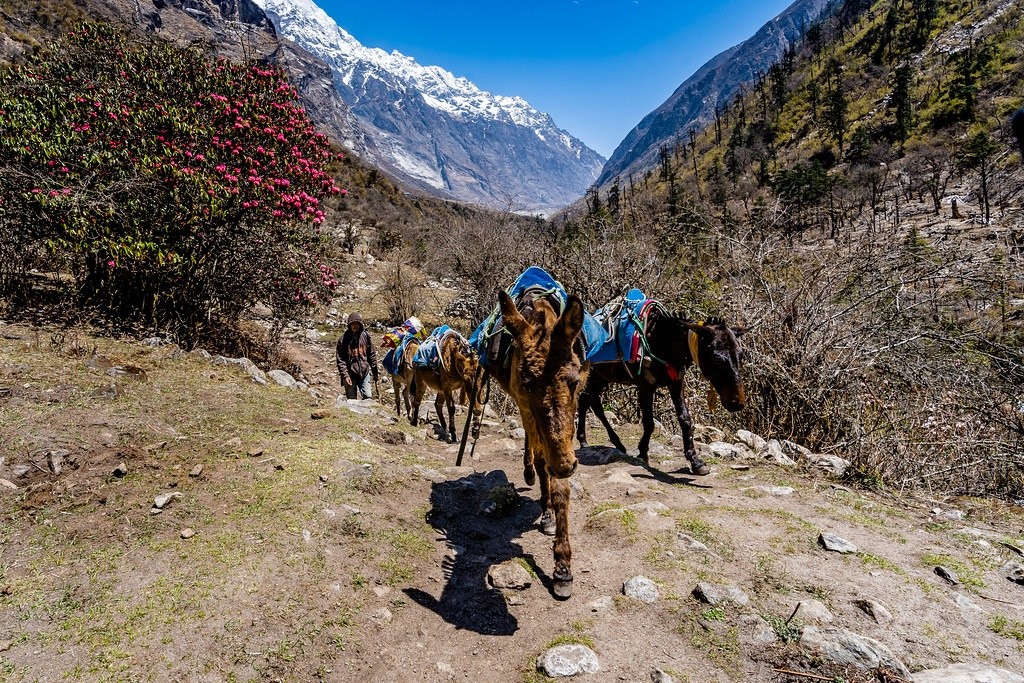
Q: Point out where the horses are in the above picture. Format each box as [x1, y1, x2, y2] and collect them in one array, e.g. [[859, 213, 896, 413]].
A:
[[383, 266, 746, 599]]
